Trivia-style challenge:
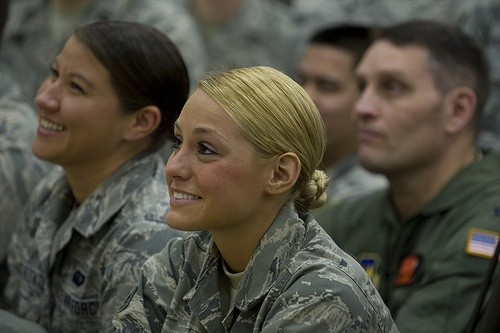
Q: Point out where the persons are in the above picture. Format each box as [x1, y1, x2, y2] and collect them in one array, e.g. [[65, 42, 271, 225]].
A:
[[298, 22, 499, 332], [0, 0, 57, 305], [110, 66, 400, 333], [4, 0, 205, 81], [0, 19, 188, 333], [298, 21, 390, 210], [195, 0, 500, 154]]
